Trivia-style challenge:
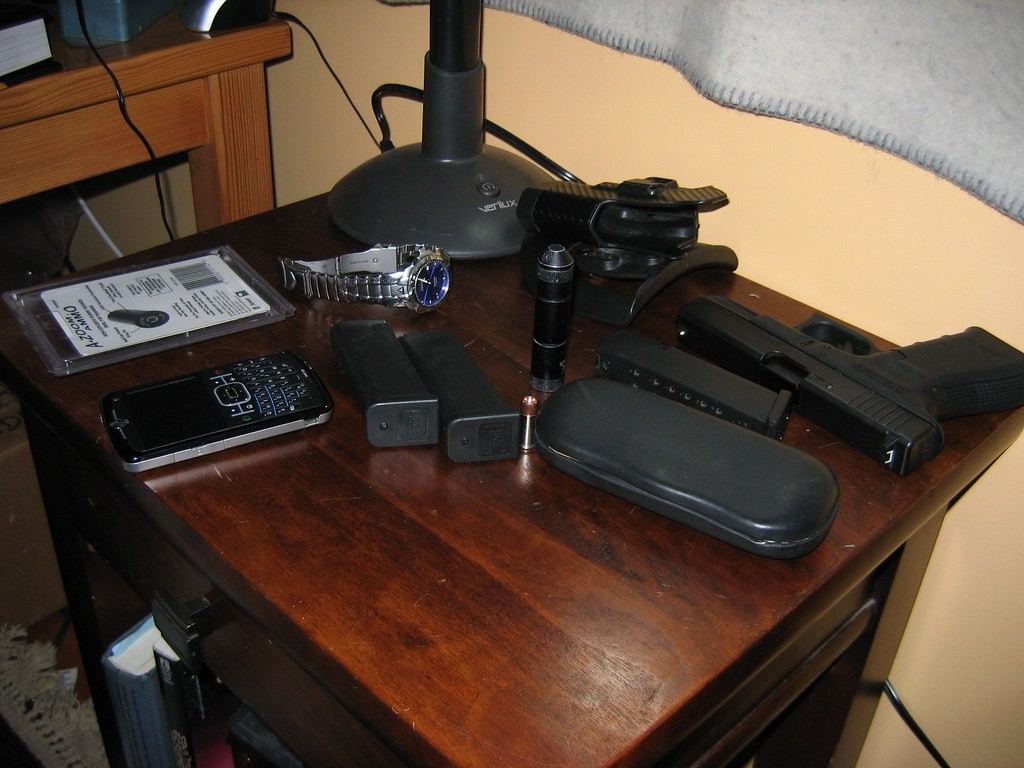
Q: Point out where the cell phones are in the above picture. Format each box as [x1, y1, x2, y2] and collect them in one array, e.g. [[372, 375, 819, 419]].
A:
[[101, 351, 335, 473]]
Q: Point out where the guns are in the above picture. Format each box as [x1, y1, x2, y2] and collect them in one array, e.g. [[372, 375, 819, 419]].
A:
[[674, 292, 1024, 479]]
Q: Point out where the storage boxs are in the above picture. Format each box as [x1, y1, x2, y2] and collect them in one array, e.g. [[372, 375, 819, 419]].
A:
[[0, 382, 73, 630]]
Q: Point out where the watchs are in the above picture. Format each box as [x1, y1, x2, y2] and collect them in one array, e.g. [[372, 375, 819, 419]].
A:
[[281, 241, 455, 313]]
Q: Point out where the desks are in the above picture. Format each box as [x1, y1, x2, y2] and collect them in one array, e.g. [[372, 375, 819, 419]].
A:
[[0, 0, 293, 233], [0, 192, 1024, 768]]
